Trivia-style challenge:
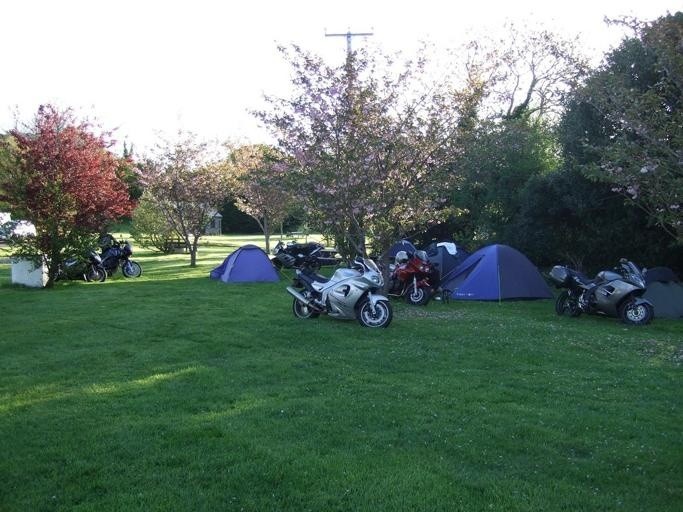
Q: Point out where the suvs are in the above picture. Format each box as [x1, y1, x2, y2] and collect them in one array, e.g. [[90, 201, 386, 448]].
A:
[[0, 219, 38, 243]]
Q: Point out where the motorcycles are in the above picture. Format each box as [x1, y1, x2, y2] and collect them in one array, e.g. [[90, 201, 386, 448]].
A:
[[369, 251, 434, 306], [548, 258, 655, 325], [285, 257, 393, 329], [269, 240, 325, 271], [52, 232, 142, 283]]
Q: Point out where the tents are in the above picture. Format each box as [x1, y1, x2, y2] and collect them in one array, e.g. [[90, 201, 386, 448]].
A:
[[379, 238, 418, 263], [438, 244, 555, 303], [420, 237, 472, 280], [636, 264, 682, 320], [208, 243, 280, 284]]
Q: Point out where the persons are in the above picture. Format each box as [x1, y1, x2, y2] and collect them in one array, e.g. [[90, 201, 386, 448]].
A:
[[96, 226, 116, 250]]
